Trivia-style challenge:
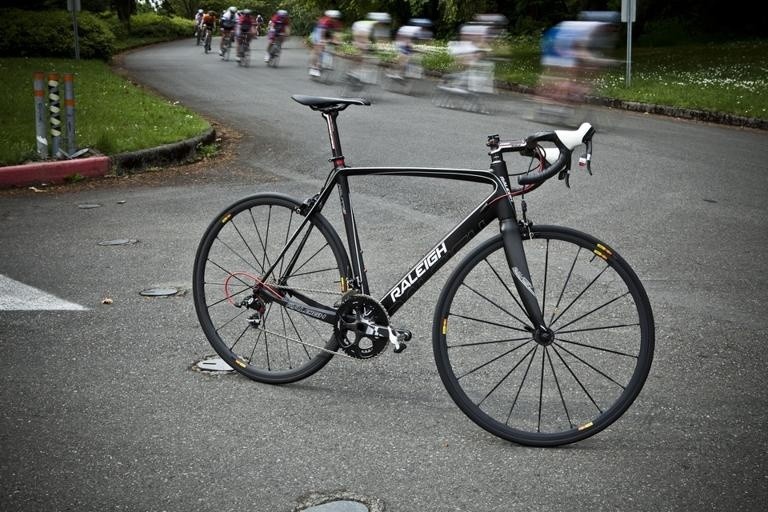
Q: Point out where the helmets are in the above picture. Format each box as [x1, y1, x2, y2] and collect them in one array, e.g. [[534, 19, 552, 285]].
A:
[[277, 10, 288, 18], [325, 9, 342, 19], [196, 6, 262, 20], [365, 12, 434, 27]]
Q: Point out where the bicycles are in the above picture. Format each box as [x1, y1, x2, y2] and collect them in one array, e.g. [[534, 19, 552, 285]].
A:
[[192, 94, 655, 447], [196, 24, 288, 68]]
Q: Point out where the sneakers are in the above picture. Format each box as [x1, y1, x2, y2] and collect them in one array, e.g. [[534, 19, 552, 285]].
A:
[[308, 67, 322, 77], [214, 50, 272, 63]]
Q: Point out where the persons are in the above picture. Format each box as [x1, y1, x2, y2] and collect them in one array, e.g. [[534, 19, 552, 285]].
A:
[[393, 16, 431, 81], [193, 6, 290, 60], [350, 10, 391, 79], [305, 8, 345, 80], [443, 11, 506, 94], [538, 9, 629, 117]]
